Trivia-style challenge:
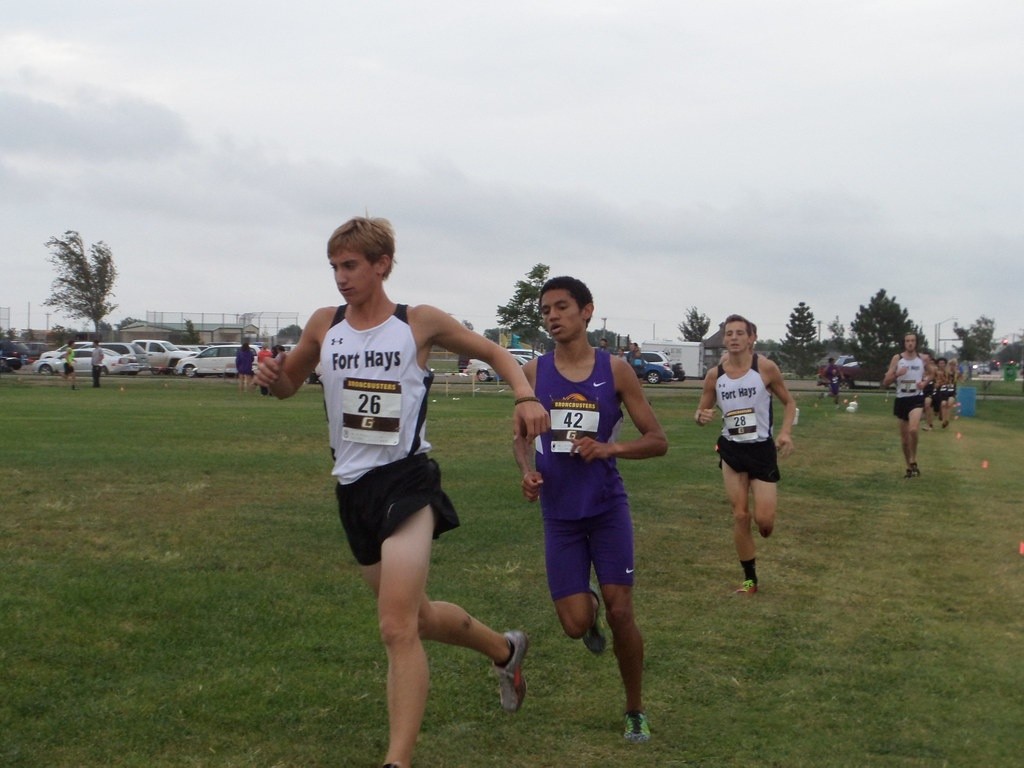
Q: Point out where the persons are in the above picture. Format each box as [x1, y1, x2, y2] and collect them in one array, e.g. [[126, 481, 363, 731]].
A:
[[822, 358, 844, 409], [598, 338, 645, 387], [236, 342, 285, 399], [693, 314, 795, 596], [508, 275, 667, 742], [90, 340, 104, 388], [884, 332, 936, 479], [921, 351, 963, 431], [249, 207, 551, 768], [55, 340, 79, 390]]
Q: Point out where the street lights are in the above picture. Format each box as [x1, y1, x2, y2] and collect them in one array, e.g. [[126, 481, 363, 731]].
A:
[[938, 317, 959, 358]]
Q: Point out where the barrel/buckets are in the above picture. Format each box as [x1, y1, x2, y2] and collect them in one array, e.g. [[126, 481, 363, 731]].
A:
[[956, 387, 975, 416], [792, 407, 799, 424]]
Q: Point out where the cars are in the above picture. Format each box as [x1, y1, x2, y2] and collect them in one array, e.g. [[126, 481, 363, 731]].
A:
[[304, 362, 322, 384], [31, 346, 140, 376], [815, 350, 891, 390], [971, 358, 1024, 374], [39, 338, 298, 375], [458, 339, 705, 383]]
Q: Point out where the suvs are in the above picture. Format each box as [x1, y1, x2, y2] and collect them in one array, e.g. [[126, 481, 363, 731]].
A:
[[174, 343, 262, 378], [0, 338, 35, 372]]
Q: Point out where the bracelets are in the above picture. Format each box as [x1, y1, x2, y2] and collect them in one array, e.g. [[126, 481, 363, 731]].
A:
[[923, 376, 929, 383], [697, 413, 701, 422], [515, 397, 540, 406], [893, 372, 898, 378]]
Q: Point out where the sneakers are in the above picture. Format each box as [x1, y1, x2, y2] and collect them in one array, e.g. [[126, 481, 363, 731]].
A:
[[904, 463, 920, 480], [733, 580, 759, 594], [623, 710, 651, 745], [922, 423, 934, 432], [941, 419, 948, 429], [488, 630, 530, 714], [582, 582, 607, 655]]
